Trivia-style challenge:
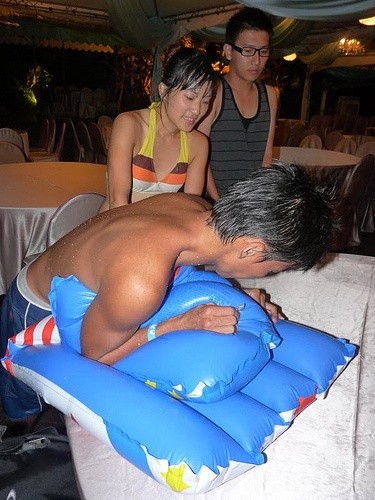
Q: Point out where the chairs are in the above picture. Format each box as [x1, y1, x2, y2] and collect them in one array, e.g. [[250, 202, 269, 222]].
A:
[[0, 86, 375, 500]]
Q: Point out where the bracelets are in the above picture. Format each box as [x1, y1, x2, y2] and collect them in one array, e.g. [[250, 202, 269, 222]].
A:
[[146, 322, 158, 343]]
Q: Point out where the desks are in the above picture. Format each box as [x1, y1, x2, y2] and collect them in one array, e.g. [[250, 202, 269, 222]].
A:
[[0, 159, 110, 299], [55, 254, 375, 500], [273, 147, 361, 186]]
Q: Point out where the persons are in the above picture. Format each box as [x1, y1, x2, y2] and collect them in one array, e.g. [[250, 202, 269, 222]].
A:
[[99, 47, 217, 215], [197, 7, 278, 206], [0, 158, 343, 438]]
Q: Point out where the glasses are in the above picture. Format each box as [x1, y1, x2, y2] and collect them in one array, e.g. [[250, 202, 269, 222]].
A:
[[232, 44, 270, 57]]
